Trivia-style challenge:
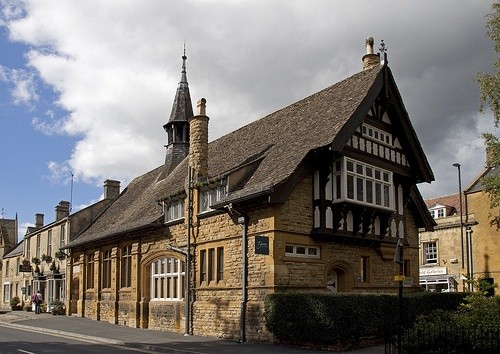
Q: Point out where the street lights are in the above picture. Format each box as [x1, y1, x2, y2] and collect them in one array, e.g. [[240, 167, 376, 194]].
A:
[[452, 163, 465, 293]]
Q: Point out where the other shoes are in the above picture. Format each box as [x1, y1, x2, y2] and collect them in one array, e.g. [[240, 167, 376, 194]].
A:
[[35, 311, 40, 314]]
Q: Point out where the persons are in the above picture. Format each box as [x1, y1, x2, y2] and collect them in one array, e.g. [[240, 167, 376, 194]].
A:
[[34, 290, 42, 314]]
